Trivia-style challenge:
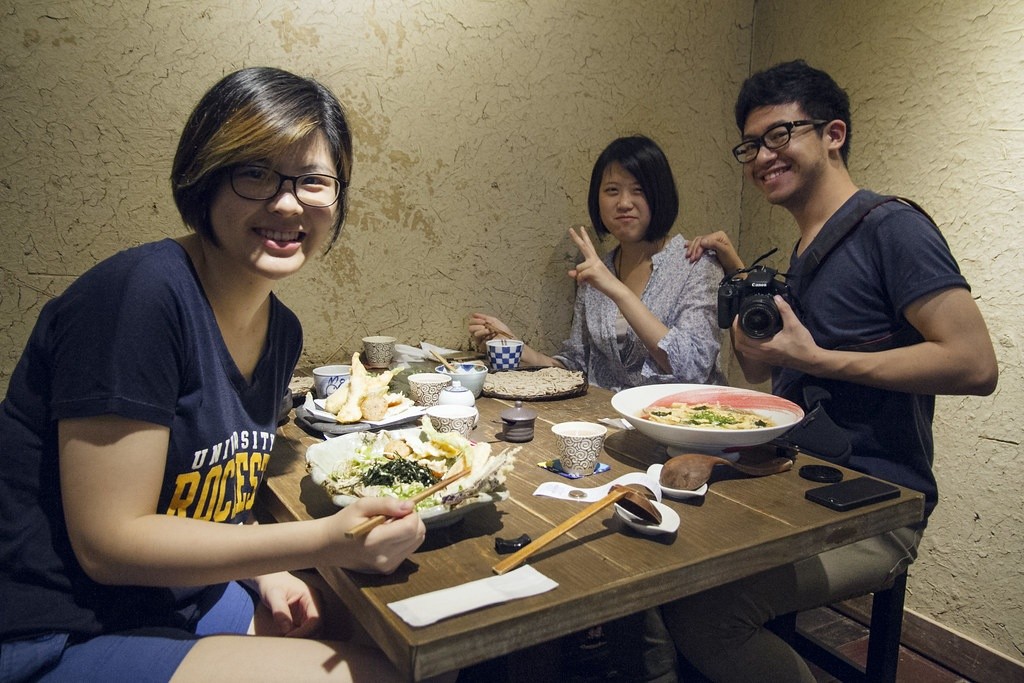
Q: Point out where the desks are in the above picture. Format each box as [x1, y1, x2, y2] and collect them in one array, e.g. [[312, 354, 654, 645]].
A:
[[253, 348, 927, 683]]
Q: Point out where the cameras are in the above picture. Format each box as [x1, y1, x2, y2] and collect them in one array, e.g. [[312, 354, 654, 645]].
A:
[[717, 270, 795, 339]]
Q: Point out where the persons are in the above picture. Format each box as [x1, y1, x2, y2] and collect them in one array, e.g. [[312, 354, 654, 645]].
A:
[[469, 133, 730, 393], [0, 68, 425, 683], [641, 59, 999, 683]]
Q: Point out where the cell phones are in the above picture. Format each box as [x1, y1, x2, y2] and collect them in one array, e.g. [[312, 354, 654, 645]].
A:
[[804, 477, 900, 512]]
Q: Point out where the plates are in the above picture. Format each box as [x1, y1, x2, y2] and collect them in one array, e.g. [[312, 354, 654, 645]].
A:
[[294, 405, 422, 435], [482, 365, 588, 400]]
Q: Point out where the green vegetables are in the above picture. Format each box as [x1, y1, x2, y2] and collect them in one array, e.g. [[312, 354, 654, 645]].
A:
[[349, 445, 464, 512], [693, 411, 739, 424]]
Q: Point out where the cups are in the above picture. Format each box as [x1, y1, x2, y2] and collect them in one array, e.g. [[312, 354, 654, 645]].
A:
[[426, 404, 477, 440], [361, 336, 397, 368], [407, 372, 452, 409], [550, 421, 607, 476]]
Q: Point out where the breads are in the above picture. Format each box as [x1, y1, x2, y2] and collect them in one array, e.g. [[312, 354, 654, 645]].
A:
[[323, 351, 407, 422]]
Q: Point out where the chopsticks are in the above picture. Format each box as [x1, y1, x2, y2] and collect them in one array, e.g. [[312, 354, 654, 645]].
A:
[[430, 350, 456, 373], [344, 466, 471, 541], [484, 322, 514, 339]]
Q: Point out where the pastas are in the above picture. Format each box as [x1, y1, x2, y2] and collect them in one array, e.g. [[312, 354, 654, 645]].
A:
[[481, 367, 585, 397]]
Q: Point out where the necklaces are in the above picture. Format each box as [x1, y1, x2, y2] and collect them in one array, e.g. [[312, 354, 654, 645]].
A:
[[619, 236, 665, 282]]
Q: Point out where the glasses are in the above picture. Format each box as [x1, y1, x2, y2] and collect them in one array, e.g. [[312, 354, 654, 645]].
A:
[[732, 119, 828, 163], [230, 164, 342, 208]]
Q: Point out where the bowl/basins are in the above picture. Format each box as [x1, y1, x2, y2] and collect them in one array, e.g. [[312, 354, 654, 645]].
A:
[[305, 428, 509, 529], [486, 339, 524, 370], [501, 401, 538, 443], [435, 362, 489, 399], [439, 380, 479, 428], [611, 383, 805, 466], [312, 365, 352, 400]]
